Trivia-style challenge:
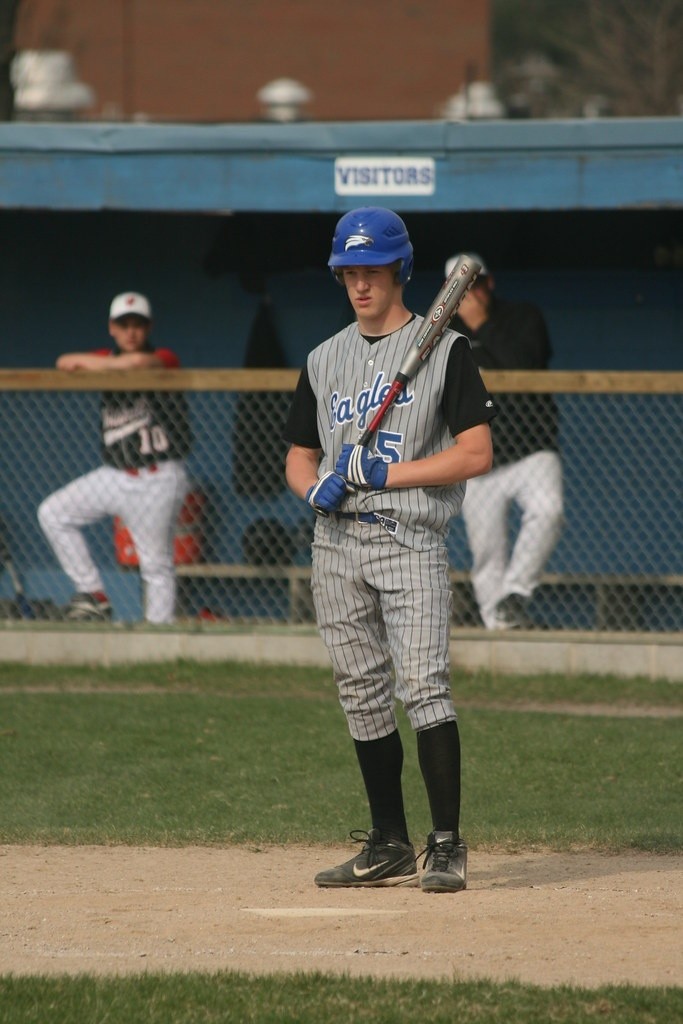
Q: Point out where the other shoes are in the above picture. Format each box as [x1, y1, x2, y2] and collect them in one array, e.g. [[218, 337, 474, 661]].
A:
[[66, 593, 113, 623], [495, 593, 526, 629]]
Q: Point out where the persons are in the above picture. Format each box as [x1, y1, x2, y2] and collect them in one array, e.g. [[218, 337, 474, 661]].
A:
[[285, 206, 495, 893], [38, 292, 194, 625], [435, 254, 568, 633]]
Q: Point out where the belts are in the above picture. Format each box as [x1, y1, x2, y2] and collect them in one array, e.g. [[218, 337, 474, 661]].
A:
[[335, 509, 379, 524]]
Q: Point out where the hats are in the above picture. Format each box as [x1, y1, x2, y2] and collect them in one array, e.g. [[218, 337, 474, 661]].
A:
[[445, 252, 487, 282], [110, 291, 152, 322]]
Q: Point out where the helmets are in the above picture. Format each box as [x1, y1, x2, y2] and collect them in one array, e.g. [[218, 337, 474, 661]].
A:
[[328, 206, 414, 287]]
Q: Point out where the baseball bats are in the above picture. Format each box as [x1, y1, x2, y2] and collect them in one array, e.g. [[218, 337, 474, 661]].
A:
[[312, 252, 484, 517], [0, 541, 36, 619]]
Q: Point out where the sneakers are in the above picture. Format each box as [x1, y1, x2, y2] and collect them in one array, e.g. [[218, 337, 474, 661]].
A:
[[416, 828, 468, 893], [314, 827, 418, 887]]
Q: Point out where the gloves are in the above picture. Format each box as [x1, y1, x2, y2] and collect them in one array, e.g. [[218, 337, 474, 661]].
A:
[[304, 470, 357, 518], [335, 444, 388, 491]]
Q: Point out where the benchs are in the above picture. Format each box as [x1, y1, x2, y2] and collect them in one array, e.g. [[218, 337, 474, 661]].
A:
[[163, 561, 683, 631]]
